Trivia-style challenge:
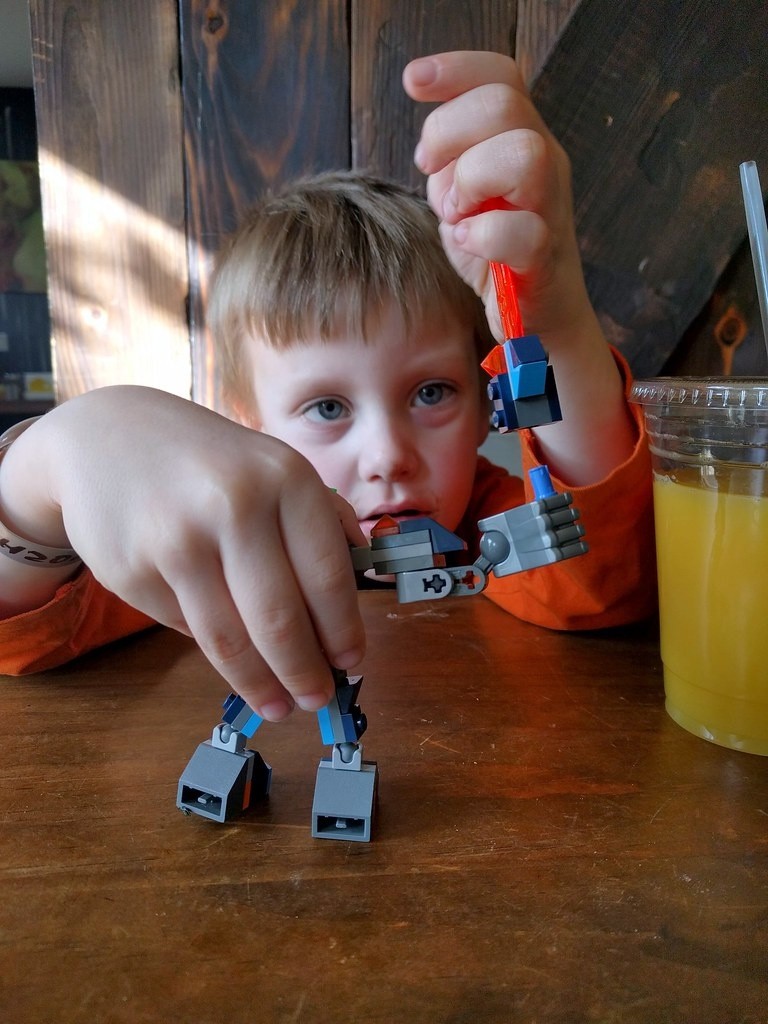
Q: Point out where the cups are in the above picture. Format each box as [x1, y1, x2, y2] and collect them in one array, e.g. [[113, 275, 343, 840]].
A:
[[631, 375, 768, 756]]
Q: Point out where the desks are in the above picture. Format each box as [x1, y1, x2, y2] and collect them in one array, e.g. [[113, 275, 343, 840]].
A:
[[0, 572, 768, 1021]]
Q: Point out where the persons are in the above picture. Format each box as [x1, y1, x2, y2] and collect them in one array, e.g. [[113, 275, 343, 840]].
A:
[[0, 51, 657, 729]]
